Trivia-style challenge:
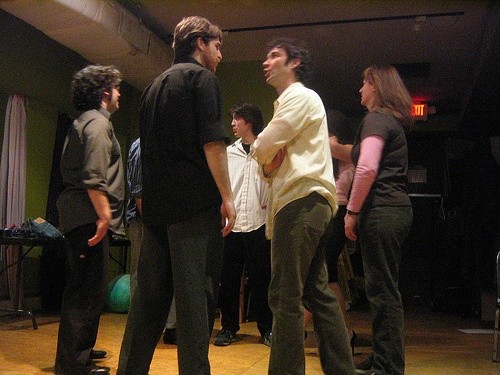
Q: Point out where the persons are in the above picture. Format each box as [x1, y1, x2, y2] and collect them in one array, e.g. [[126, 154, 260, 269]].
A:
[[55, 65, 127, 375], [125, 64, 414, 375], [114, 14, 238, 375], [250, 38, 356, 375]]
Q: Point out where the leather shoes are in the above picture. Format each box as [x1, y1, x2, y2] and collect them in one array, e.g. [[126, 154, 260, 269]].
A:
[[91, 366, 110, 375], [90, 350, 106, 358]]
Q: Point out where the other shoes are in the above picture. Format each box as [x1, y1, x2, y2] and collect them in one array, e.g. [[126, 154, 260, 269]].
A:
[[354, 359, 384, 375], [259, 337, 271, 345], [214, 328, 239, 346], [163, 328, 177, 345], [349, 330, 358, 355]]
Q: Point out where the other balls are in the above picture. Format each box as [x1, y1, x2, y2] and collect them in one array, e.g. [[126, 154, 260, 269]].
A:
[[105, 273, 132, 315]]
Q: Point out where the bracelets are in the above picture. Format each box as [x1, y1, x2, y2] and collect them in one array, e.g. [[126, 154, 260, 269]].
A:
[[346, 209, 358, 215]]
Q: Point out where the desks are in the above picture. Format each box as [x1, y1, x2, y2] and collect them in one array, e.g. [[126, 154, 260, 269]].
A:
[[0, 237, 132, 330]]
